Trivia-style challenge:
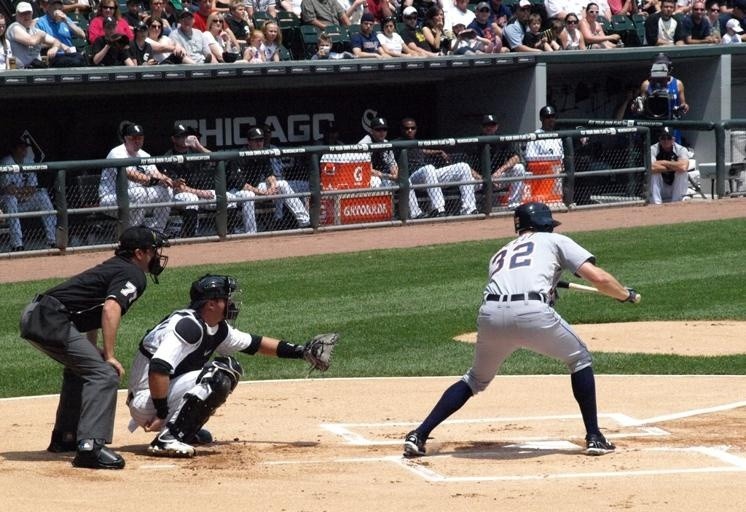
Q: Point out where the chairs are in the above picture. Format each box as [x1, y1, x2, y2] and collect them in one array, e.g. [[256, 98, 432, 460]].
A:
[[0, 0, 684, 59]]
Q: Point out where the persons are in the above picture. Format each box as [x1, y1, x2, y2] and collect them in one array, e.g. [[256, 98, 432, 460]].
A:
[[1, 140, 57, 249], [126, 276, 340, 459], [355, 114, 525, 219], [525, 105, 610, 200], [98, 124, 311, 237], [20, 226, 170, 470], [615, 54, 689, 203], [403, 202, 640, 456], [0, 0, 746, 69]]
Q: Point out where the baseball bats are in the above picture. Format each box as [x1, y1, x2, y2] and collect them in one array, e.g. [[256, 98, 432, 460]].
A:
[[557, 281, 641, 303]]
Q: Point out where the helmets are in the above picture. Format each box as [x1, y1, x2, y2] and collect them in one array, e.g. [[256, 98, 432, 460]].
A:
[[515, 202, 561, 232], [190, 275, 241, 326], [121, 225, 169, 275]]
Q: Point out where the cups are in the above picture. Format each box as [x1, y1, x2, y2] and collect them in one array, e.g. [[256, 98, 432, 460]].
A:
[[183, 136, 196, 147], [9, 58, 17, 69]]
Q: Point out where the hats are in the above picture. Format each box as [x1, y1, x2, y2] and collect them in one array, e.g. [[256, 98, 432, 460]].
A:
[[121, 123, 143, 136], [403, 6, 417, 16], [172, 124, 188, 136], [246, 126, 263, 141], [102, 15, 116, 26], [540, 105, 555, 119], [17, 2, 32, 13], [371, 117, 387, 129], [179, 7, 193, 20], [482, 114, 497, 125], [519, 0, 532, 9], [659, 127, 672, 136], [475, 2, 490, 11], [727, 19, 744, 33]]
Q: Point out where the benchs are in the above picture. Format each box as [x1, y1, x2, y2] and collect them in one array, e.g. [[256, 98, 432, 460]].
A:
[[0, 122, 624, 249]]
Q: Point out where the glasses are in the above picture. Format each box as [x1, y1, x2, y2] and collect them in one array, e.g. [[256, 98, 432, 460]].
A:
[[213, 19, 222, 23], [568, 18, 577, 24]]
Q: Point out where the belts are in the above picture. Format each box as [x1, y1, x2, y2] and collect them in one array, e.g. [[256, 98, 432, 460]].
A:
[[33, 293, 44, 301], [485, 294, 544, 301]]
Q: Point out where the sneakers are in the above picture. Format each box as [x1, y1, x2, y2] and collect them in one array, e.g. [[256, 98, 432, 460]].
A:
[[147, 435, 196, 457], [51, 430, 78, 450], [585, 433, 617, 454], [72, 447, 124, 468], [402, 432, 426, 455], [184, 432, 211, 447]]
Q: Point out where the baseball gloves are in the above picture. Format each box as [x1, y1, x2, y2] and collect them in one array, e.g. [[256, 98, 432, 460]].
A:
[[304, 333, 338, 372]]
